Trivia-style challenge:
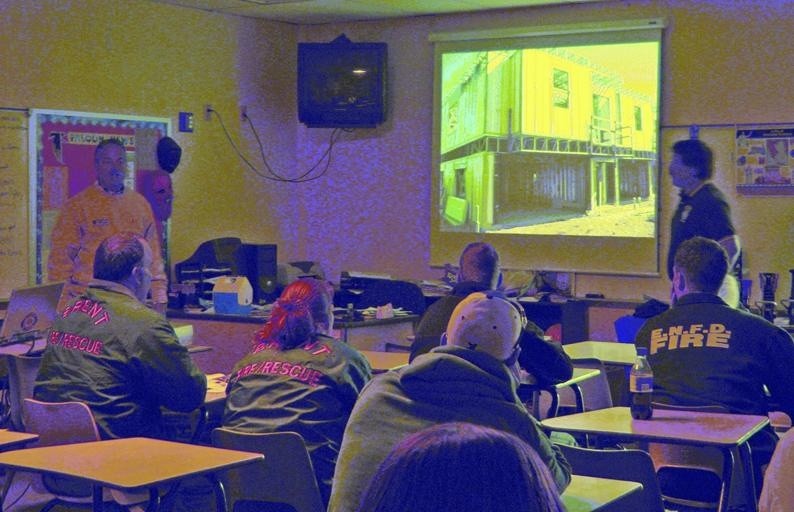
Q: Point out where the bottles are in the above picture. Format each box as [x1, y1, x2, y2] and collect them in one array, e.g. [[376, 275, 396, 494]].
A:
[[628, 348, 656, 419]]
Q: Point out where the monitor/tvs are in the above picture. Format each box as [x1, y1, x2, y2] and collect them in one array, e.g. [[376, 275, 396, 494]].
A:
[[174, 236, 244, 299], [296, 40, 390, 128]]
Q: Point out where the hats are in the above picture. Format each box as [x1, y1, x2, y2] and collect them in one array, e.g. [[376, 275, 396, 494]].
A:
[[447, 291, 527, 361]]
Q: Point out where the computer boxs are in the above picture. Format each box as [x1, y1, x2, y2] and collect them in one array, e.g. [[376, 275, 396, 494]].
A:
[[244, 243, 278, 304]]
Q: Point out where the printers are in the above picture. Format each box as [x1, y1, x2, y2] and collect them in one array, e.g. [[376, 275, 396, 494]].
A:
[[277, 260, 326, 288]]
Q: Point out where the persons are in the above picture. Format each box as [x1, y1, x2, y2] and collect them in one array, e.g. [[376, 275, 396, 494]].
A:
[[32, 233, 217, 512], [220, 276, 374, 512], [356, 421, 569, 512], [634, 236, 794, 512], [667, 139, 743, 305], [45, 137, 168, 316], [326, 290, 572, 512], [408, 241, 573, 388]]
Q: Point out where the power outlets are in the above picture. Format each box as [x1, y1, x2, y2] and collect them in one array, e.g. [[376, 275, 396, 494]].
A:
[[238, 103, 247, 121], [202, 103, 212, 121]]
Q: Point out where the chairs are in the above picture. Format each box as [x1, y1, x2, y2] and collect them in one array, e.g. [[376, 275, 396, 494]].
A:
[[0, 272, 791, 511]]
